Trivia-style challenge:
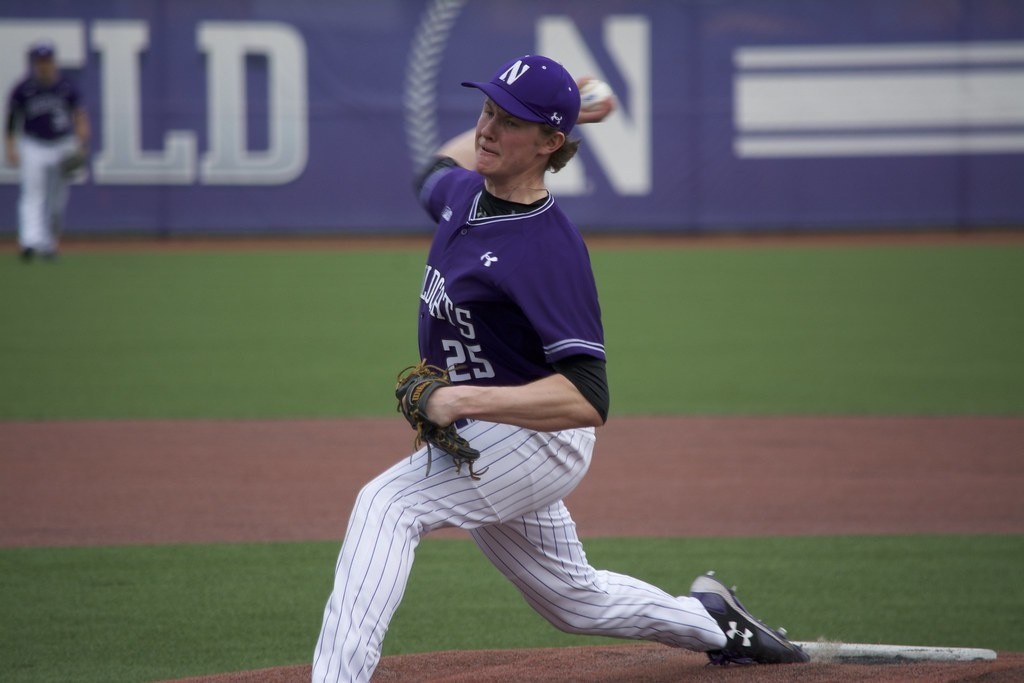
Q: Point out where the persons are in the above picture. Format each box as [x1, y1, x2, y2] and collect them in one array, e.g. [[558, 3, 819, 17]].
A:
[[5, 44, 92, 261], [313, 56, 810, 683]]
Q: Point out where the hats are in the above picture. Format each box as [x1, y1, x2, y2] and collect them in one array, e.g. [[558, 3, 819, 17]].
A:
[[461, 54, 581, 136], [30, 46, 52, 62]]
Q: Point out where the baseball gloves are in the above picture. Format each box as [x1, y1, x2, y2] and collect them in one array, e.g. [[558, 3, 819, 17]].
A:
[[395, 365, 482, 462]]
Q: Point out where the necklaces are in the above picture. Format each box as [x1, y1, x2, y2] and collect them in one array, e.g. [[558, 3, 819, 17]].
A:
[[506, 185, 548, 200]]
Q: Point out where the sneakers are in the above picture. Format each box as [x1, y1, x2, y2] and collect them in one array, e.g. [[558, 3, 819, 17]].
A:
[[688, 571, 811, 665]]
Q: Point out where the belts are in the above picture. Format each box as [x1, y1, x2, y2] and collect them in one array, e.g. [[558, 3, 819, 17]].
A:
[[455, 418, 475, 429]]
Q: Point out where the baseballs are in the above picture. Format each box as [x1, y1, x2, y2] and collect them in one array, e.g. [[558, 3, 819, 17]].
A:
[[578, 78, 614, 110]]
